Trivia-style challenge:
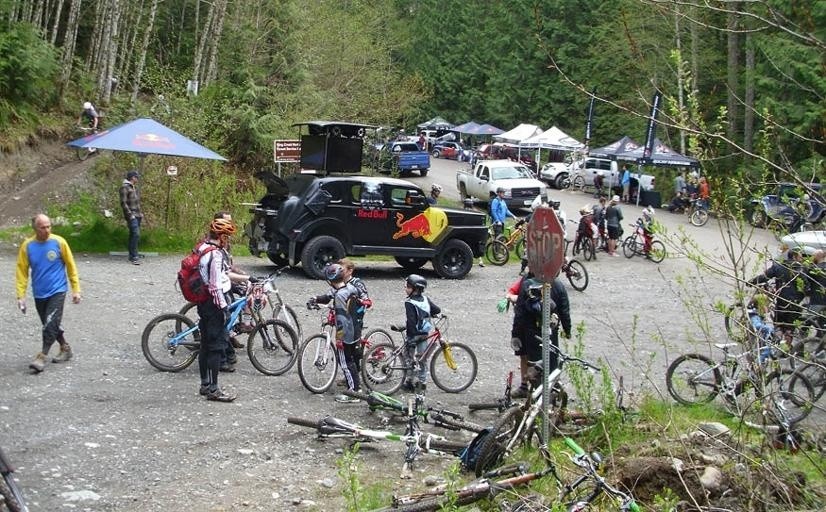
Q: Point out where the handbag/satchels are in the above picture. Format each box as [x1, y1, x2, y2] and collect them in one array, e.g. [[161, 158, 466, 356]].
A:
[[415, 316, 434, 335]]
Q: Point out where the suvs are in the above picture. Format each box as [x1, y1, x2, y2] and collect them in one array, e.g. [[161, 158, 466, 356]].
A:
[[240, 166, 490, 284], [747, 181, 826, 229]]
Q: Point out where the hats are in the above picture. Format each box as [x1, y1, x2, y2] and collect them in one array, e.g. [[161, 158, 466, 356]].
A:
[[496, 187, 506, 194], [405, 274, 427, 289], [324, 264, 345, 282], [126, 170, 141, 179], [209, 219, 236, 236], [431, 183, 443, 193]]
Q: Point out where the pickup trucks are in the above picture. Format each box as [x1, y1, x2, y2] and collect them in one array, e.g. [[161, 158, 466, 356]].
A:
[[368, 129, 659, 214]]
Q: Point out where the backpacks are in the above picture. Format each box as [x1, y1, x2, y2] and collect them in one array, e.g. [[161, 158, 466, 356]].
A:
[[177, 242, 217, 304]]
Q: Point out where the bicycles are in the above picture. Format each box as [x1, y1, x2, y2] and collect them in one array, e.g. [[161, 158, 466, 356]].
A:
[[75, 125, 102, 163], [771, 210, 812, 241], [686, 195, 710, 227], [0, 444, 27, 512], [665, 297, 826, 432], [483, 205, 668, 291]]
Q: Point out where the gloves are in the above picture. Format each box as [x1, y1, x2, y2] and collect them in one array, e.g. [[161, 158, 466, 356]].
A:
[[307, 297, 317, 311], [497, 297, 509, 314], [220, 306, 232, 327], [511, 337, 522, 352]]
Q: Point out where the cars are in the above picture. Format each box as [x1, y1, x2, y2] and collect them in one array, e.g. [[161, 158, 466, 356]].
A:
[[778, 228, 826, 265]]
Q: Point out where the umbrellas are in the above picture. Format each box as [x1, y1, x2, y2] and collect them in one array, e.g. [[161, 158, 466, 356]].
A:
[[64, 115, 229, 192]]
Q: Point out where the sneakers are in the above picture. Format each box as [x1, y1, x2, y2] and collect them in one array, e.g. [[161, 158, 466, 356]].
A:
[[52, 344, 72, 364], [400, 380, 414, 393], [200, 384, 211, 396], [226, 355, 237, 364], [230, 337, 245, 349], [207, 390, 236, 403], [29, 353, 47, 374], [219, 364, 235, 373], [238, 322, 257, 334], [510, 388, 530, 399], [415, 381, 427, 392], [334, 392, 362, 405]]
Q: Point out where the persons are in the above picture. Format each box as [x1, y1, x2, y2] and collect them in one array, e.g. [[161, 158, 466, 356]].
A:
[[119, 169, 144, 265], [796, 248, 826, 358], [426, 183, 443, 204], [744, 248, 805, 346], [418, 133, 426, 152], [491, 162, 710, 258], [15, 214, 81, 373], [307, 257, 372, 387], [505, 255, 530, 398], [196, 218, 237, 402], [510, 273, 572, 376], [76, 101, 100, 134], [322, 261, 363, 404], [214, 211, 256, 373], [726, 293, 782, 407], [401, 273, 442, 393]]
